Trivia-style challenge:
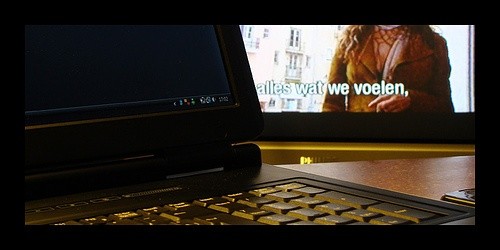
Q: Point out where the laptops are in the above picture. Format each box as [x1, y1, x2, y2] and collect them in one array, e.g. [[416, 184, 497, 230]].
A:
[[24, 24, 476, 225]]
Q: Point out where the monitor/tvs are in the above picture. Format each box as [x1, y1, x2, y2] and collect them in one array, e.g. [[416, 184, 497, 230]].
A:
[[238, 25, 475, 143]]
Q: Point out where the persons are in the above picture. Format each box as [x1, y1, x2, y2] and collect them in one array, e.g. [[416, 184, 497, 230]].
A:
[[322, 25, 455, 113]]
